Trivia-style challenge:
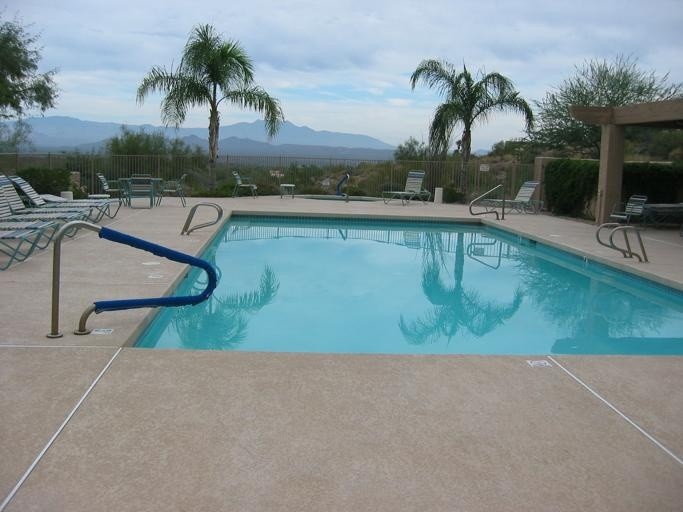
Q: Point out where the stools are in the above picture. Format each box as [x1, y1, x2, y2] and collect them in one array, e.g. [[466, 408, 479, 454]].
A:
[[280, 184, 295, 199]]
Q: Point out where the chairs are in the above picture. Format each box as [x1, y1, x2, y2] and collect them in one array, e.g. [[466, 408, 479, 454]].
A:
[[232, 171, 257, 199], [608, 195, 647, 230], [484, 181, 544, 215], [382, 170, 431, 206], [0, 175, 187, 270], [639, 203, 683, 229]]
